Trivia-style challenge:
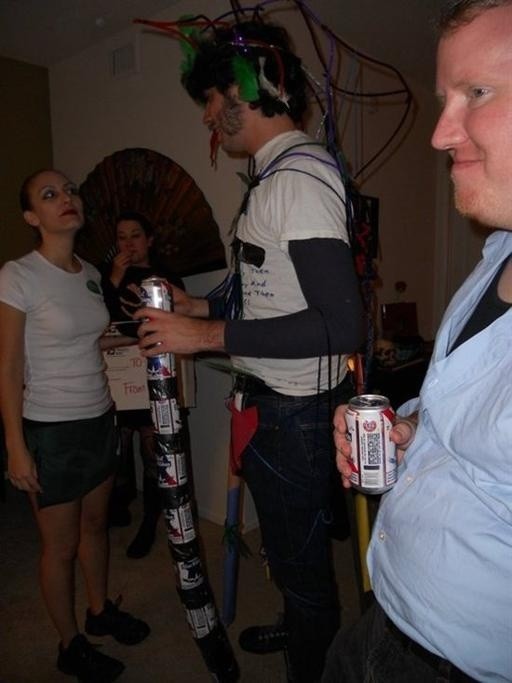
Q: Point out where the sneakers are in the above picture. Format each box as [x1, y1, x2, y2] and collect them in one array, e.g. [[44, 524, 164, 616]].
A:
[[56, 634, 126, 683], [237, 624, 289, 655], [108, 507, 158, 559], [84, 599, 150, 646]]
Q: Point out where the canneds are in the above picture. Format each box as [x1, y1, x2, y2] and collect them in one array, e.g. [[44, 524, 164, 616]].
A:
[[346, 394, 397, 495], [140, 277, 242, 683]]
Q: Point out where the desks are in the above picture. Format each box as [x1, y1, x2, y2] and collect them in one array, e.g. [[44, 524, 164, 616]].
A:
[[179, 356, 259, 535]]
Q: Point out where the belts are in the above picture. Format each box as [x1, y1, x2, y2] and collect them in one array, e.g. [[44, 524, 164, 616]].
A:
[[385, 613, 483, 683], [232, 375, 278, 397]]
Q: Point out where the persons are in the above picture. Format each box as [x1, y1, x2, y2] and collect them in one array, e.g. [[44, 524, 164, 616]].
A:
[[98, 213, 187, 558], [318, 0, 512, 683], [129, 22, 368, 683], [0, 168, 152, 683]]
